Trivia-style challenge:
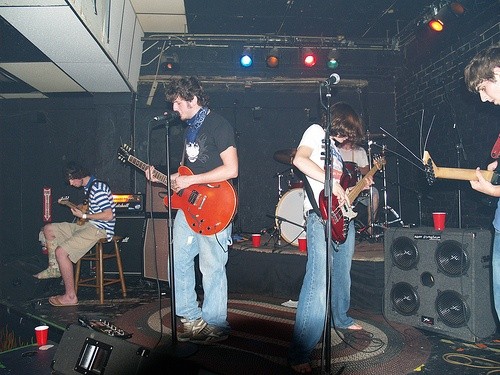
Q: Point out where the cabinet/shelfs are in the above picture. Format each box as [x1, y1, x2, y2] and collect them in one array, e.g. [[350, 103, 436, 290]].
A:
[[0, 0, 144, 94]]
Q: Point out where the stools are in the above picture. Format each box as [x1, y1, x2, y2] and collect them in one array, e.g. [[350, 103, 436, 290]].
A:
[[75, 235, 128, 306]]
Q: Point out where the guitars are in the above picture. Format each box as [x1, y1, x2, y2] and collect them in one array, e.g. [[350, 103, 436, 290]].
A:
[[422, 148, 499, 186], [57, 196, 89, 225], [117, 143, 237, 235], [318, 153, 387, 242]]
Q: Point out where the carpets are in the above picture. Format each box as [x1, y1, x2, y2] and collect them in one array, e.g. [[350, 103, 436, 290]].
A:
[[113, 292, 432, 375]]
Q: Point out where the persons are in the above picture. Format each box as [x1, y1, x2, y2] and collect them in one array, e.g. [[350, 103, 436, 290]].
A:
[[337, 139, 380, 234], [32, 162, 116, 306], [463, 41, 500, 321], [145, 78, 238, 341], [293, 102, 374, 350]]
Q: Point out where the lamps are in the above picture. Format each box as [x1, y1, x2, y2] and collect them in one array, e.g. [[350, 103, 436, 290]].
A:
[[162, 46, 181, 75], [239, 46, 256, 70], [266, 48, 282, 68], [326, 50, 343, 72], [428, 6, 450, 32], [300, 48, 317, 68]]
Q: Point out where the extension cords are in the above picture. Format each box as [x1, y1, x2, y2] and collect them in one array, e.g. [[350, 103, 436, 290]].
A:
[[147, 81, 158, 105]]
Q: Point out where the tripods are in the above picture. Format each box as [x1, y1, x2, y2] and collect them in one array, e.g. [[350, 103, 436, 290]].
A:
[[355, 131, 403, 242]]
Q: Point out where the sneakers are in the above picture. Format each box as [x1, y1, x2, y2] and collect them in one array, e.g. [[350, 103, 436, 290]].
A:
[[190, 325, 228, 345], [178, 318, 207, 342]]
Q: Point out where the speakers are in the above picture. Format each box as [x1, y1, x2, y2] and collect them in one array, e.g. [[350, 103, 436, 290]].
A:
[[90, 181, 176, 286], [49, 323, 159, 375], [383, 225, 497, 342]]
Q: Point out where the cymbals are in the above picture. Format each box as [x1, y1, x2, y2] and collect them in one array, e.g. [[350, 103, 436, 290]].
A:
[[274, 149, 296, 165], [364, 133, 386, 141], [376, 151, 398, 156]]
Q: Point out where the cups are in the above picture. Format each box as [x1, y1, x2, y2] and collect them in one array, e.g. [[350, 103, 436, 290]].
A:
[[298, 237, 307, 252], [432, 212, 447, 230], [251, 234, 261, 247], [35, 325, 49, 346]]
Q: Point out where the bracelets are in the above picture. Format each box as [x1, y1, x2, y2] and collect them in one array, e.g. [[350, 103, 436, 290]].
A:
[[82, 214, 86, 219]]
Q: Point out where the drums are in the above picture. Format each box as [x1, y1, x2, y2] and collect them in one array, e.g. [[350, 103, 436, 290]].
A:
[[274, 188, 313, 246], [340, 161, 358, 191]]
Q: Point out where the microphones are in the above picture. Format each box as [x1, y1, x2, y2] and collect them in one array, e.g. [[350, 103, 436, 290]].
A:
[[322, 73, 341, 86], [155, 111, 180, 121]]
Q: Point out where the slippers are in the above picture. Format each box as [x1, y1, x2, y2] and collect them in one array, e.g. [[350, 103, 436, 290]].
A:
[[49, 295, 79, 307]]
